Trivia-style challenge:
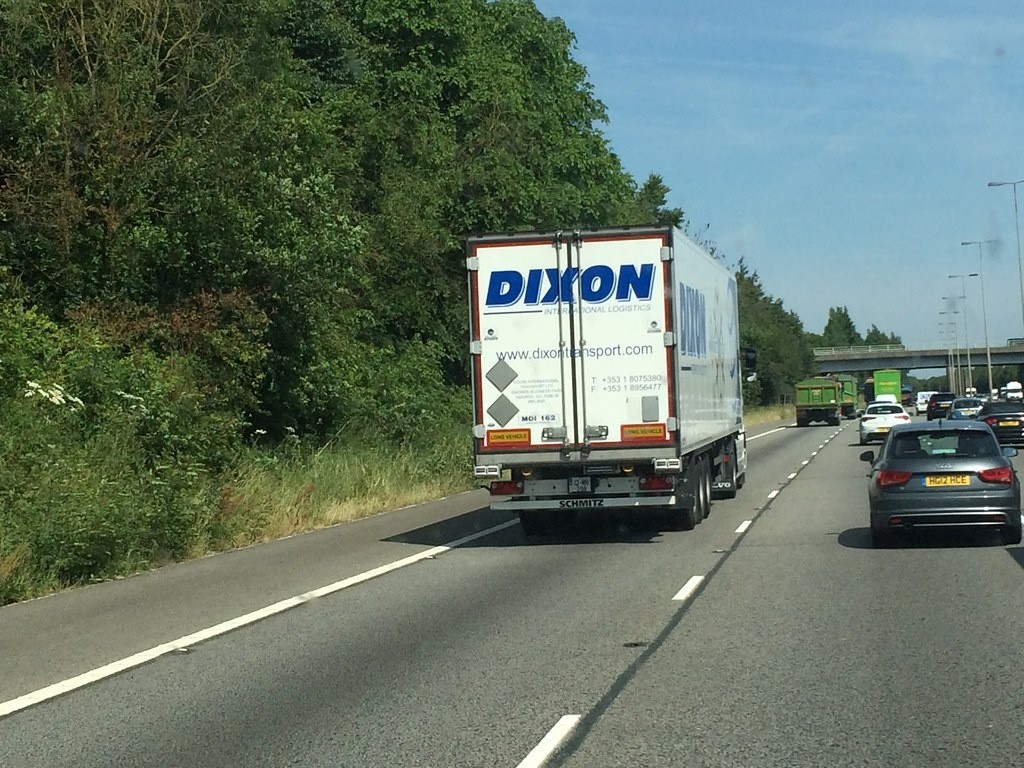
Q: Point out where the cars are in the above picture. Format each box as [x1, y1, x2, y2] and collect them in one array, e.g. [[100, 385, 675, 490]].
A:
[[925, 394, 1024, 444], [860, 401, 912, 445], [860, 417, 1022, 548]]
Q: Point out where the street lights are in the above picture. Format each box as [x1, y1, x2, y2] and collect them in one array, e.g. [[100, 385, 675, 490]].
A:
[[988, 180, 1024, 328], [961, 240, 997, 403], [942, 295, 966, 398], [938, 311, 959, 398], [948, 274, 978, 397]]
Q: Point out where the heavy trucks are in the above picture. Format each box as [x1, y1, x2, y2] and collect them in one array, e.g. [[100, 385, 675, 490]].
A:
[[795, 375, 858, 427], [873, 370, 901, 404], [466, 223, 747, 535]]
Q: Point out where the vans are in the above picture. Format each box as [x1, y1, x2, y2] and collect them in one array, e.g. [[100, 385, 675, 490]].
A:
[[966, 388, 977, 397], [915, 391, 938, 416], [992, 381, 1023, 403]]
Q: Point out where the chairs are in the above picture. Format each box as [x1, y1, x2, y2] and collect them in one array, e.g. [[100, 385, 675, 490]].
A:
[[956, 437, 979, 455], [898, 438, 927, 457]]
